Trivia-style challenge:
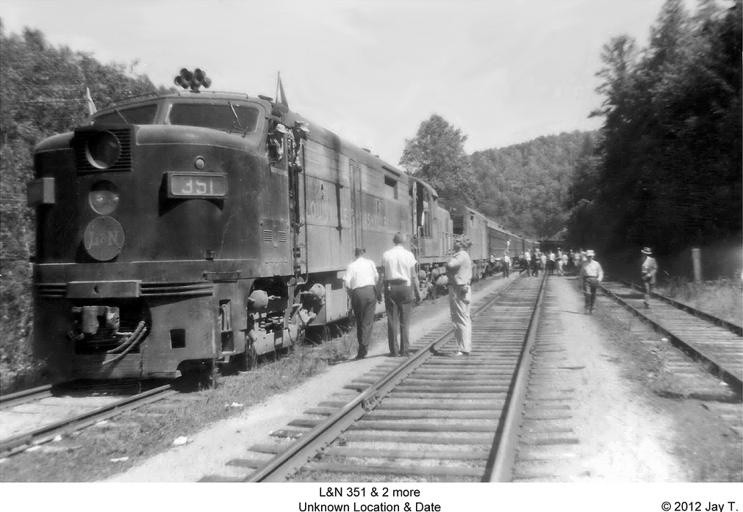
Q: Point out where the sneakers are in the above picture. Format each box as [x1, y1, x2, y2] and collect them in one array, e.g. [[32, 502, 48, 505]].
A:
[[388, 351, 414, 357], [355, 353, 365, 361]]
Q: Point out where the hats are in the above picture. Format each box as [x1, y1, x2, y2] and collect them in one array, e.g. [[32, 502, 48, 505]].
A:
[[453, 351, 468, 358], [456, 238, 472, 250], [641, 247, 654, 255], [586, 249, 596, 257]]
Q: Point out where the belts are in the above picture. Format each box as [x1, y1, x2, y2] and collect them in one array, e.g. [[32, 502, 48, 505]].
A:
[[584, 276, 598, 278]]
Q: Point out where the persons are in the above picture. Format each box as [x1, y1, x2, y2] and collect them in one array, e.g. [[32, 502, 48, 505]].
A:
[[636, 247, 658, 309], [501, 247, 586, 278], [446, 236, 472, 357], [580, 250, 604, 315], [345, 248, 382, 359], [382, 232, 422, 357]]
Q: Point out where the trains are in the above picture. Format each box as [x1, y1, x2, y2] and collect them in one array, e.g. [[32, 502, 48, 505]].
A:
[[25, 62, 568, 390]]
